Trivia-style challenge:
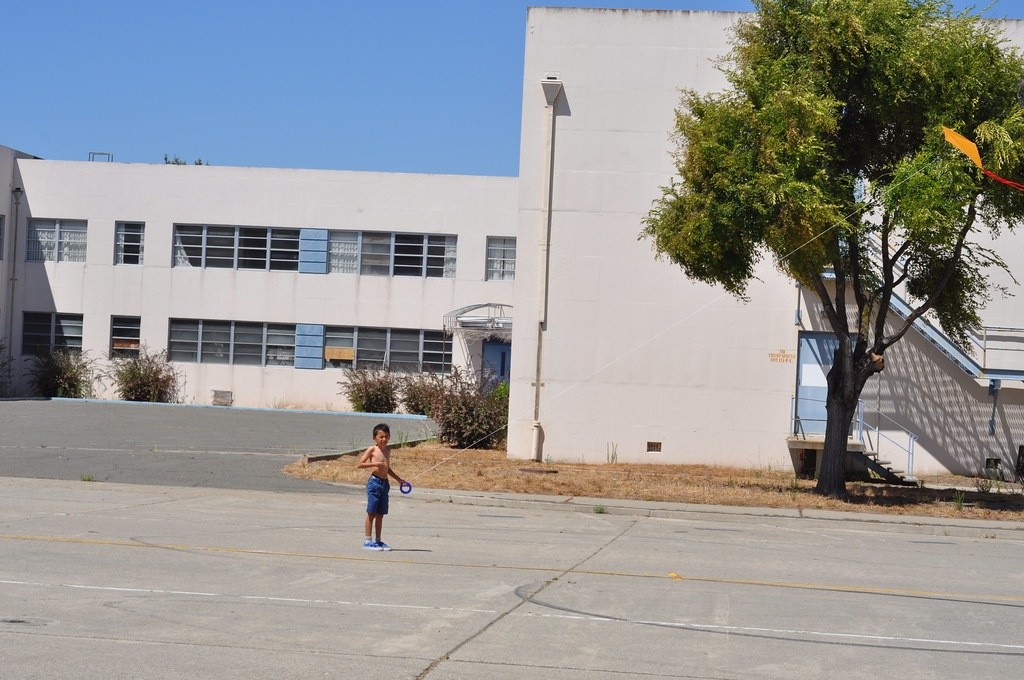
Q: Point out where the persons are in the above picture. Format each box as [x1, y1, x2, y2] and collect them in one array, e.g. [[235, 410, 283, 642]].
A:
[[358, 423, 405, 551]]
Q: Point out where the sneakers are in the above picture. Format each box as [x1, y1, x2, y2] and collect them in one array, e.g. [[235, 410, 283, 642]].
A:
[[363, 540, 382, 550], [375, 542, 392, 550]]
